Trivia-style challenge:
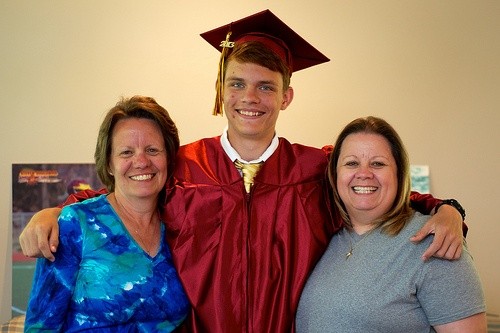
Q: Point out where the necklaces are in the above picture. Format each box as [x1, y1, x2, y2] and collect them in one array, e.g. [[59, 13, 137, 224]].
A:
[[346, 226, 380, 257], [114, 193, 159, 256]]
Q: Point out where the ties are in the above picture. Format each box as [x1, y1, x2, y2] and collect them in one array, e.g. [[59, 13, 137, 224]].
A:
[[238, 162, 259, 192]]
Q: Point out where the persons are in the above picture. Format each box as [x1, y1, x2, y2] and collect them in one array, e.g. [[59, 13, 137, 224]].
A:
[[23, 95, 190, 333], [295, 116, 487, 333], [19, 37, 469, 333]]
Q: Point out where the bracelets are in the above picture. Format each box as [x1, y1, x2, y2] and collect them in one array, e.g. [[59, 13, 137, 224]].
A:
[[434, 198, 466, 222]]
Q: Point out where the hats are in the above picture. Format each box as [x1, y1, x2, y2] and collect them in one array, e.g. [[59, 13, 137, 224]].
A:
[[199, 8, 331, 116]]
